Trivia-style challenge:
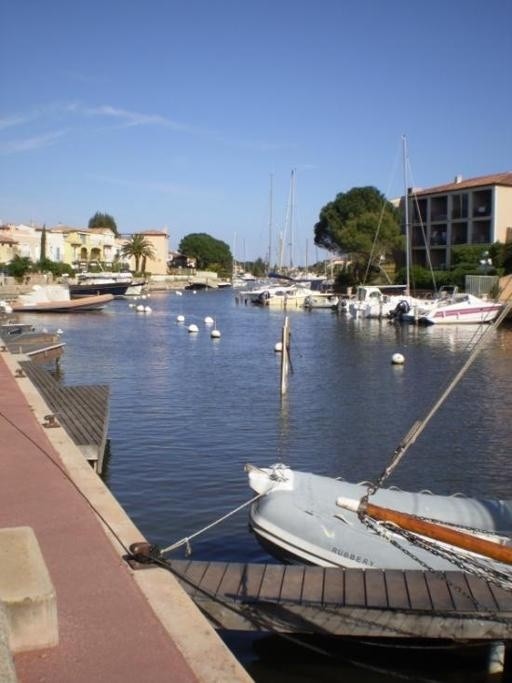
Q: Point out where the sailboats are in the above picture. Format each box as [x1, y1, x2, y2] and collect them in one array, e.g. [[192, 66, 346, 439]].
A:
[[232, 132, 503, 325]]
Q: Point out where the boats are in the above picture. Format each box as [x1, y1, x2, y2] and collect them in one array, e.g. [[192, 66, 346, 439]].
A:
[[244, 462, 512, 571], [15, 306, 115, 333], [68, 259, 149, 295], [0, 282, 115, 312], [218, 281, 232, 288]]
